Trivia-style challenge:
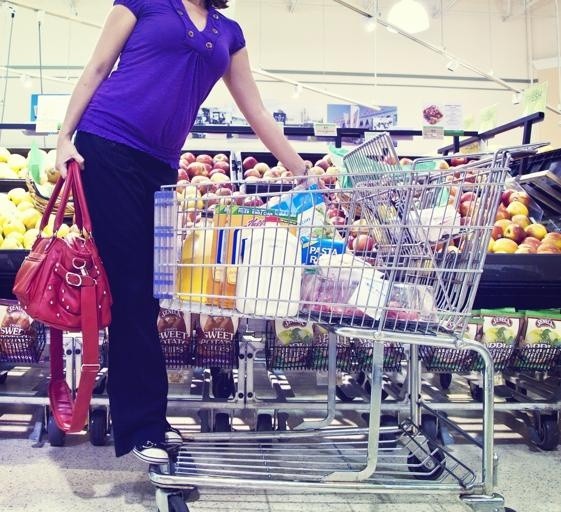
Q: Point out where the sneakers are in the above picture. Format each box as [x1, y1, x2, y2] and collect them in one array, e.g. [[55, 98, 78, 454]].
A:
[[131, 430, 183, 465]]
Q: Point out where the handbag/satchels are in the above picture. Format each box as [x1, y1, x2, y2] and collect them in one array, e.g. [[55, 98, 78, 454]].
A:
[[12, 158, 113, 332]]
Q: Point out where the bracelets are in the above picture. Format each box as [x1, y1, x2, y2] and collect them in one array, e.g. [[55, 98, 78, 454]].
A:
[[299, 165, 309, 185]]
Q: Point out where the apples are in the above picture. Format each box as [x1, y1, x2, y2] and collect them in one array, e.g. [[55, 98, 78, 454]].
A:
[[173, 151, 561, 254], [0, 188, 89, 249], [0, 147, 30, 179]]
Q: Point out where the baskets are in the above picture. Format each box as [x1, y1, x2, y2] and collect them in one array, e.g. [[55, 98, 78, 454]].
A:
[[265, 317, 561, 374], [0, 320, 46, 364], [156, 330, 239, 370], [158, 338, 561, 374], [25, 176, 76, 218]]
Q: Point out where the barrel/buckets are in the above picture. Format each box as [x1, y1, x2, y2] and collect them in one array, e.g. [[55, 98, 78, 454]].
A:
[[175, 211, 213, 304], [236, 217, 301, 318]]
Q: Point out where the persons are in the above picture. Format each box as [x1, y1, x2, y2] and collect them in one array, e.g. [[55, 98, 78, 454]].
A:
[[55, 0, 316, 463]]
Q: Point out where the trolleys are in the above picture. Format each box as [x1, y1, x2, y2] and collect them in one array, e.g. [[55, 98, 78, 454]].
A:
[[148, 132, 551, 512]]
